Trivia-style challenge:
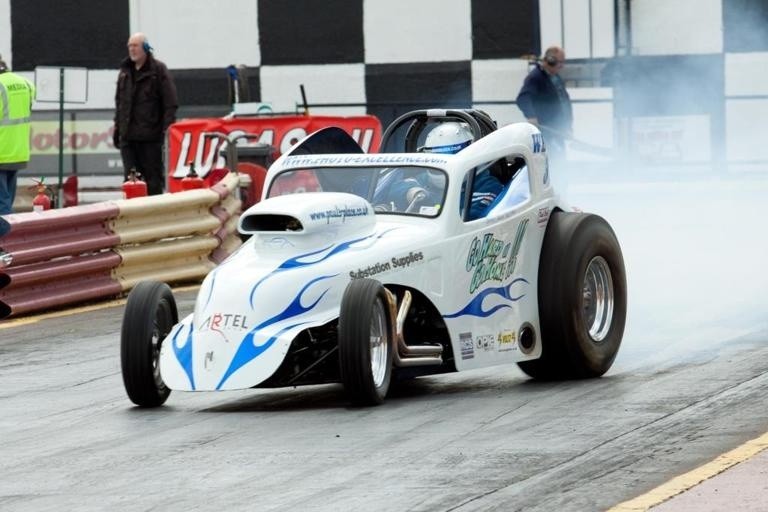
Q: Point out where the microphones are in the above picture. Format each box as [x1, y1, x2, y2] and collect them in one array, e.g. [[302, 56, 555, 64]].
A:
[[299, 83, 308, 107], [228, 62, 241, 85]]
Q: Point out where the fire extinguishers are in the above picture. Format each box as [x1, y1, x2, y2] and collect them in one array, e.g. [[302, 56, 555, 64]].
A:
[[181, 161, 203, 191], [122, 168, 147, 199], [27, 179, 54, 212]]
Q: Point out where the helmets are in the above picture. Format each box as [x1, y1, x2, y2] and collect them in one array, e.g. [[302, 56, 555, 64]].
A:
[[422, 123, 474, 190]]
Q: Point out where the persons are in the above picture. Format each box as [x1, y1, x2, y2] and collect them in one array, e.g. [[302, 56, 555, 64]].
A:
[[385, 121, 505, 220], [0, 54, 36, 236], [514, 46, 572, 183], [112, 31, 177, 194]]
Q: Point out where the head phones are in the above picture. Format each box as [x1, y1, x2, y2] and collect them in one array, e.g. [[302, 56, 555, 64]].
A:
[[144, 40, 154, 52], [535, 54, 557, 67]]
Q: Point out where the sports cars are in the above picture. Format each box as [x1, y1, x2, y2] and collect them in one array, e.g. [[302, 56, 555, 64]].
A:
[[119, 106, 629, 411]]
